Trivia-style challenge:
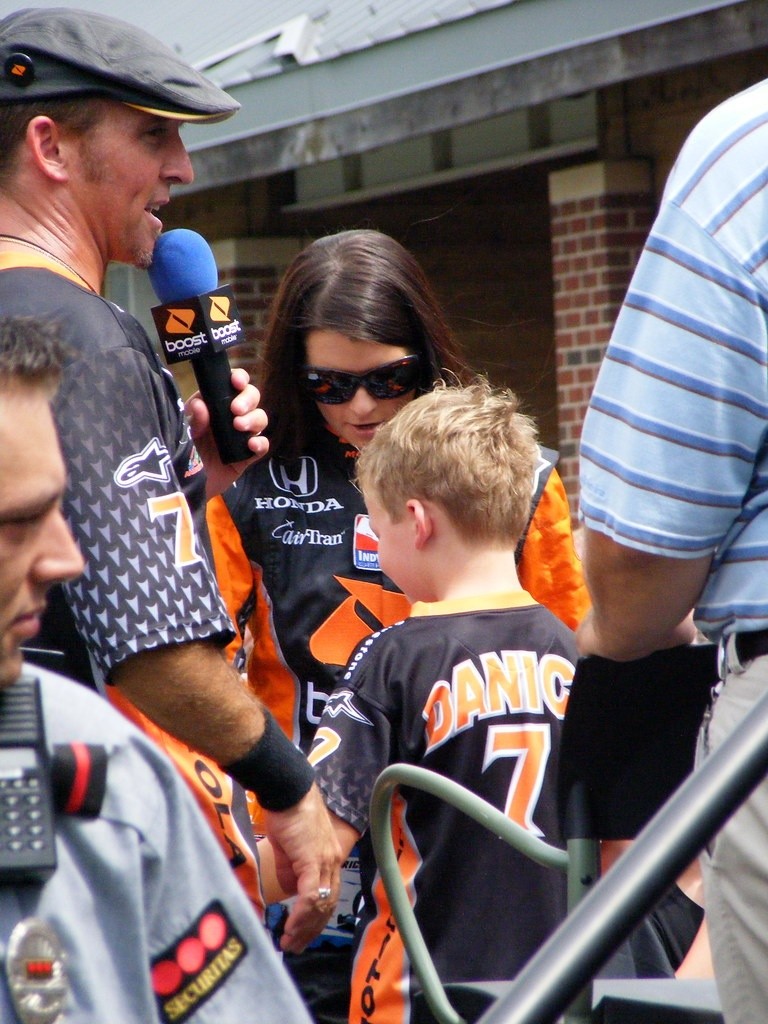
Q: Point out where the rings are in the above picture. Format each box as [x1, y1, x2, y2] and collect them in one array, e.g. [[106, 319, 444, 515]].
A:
[[319, 889, 330, 898]]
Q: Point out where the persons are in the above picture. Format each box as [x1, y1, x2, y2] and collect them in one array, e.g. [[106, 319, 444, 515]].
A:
[[575, 78, 768, 1024], [0, 6, 344, 953], [0, 313, 314, 1024], [110, 230, 594, 967], [256, 383, 586, 1024]]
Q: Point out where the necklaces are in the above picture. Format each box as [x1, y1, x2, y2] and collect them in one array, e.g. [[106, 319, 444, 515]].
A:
[[0, 234, 99, 295]]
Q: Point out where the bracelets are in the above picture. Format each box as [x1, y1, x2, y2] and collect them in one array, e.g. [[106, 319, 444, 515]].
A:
[[218, 701, 316, 813]]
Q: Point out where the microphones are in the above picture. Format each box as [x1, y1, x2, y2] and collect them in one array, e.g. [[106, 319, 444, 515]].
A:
[[148, 229, 255, 464]]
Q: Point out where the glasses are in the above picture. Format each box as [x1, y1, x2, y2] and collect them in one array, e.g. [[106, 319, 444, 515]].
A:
[[297, 353, 437, 404]]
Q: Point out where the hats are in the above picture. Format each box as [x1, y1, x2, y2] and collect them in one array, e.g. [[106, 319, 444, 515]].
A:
[[0, 8, 241, 124]]
